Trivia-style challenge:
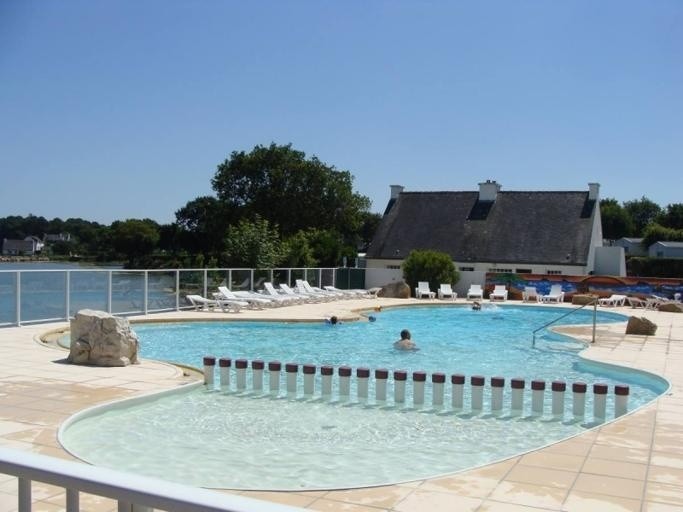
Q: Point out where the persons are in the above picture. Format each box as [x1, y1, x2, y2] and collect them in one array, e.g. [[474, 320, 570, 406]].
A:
[[330, 315, 338, 324], [392, 328, 418, 348], [472, 301, 481, 310]]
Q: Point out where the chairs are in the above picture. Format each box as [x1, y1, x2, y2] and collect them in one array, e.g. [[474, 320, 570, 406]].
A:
[[597, 292, 681, 310], [185, 277, 381, 314], [414, 281, 565, 304]]
